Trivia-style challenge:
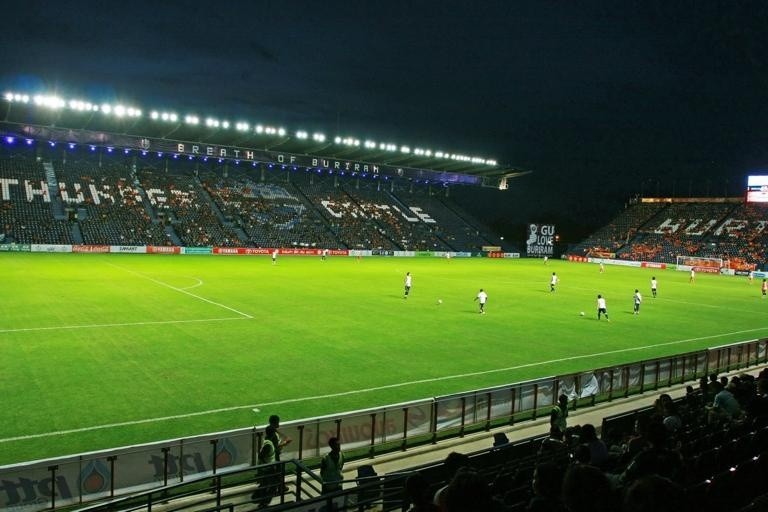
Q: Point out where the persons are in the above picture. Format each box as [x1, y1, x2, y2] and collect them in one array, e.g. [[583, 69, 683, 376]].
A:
[[586, 205, 767, 273], [446, 251, 449, 260], [760, 279, 767, 296], [0, 202, 15, 245], [252, 414, 296, 500], [596, 294, 611, 322], [418, 368, 768, 512], [402, 270, 413, 300], [82, 176, 400, 265], [319, 437, 347, 494], [688, 269, 696, 284], [548, 273, 559, 293], [544, 255, 548, 264], [257, 426, 282, 507], [650, 275, 658, 298], [632, 288, 642, 314], [474, 289, 488, 316]]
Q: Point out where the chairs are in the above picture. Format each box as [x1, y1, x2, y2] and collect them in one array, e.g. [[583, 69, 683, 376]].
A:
[[337, 365, 767, 511], [0, 152, 766, 276]]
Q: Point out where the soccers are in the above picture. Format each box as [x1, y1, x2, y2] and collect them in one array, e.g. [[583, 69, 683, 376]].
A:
[[580, 312, 584, 316], [438, 300, 442, 304]]
